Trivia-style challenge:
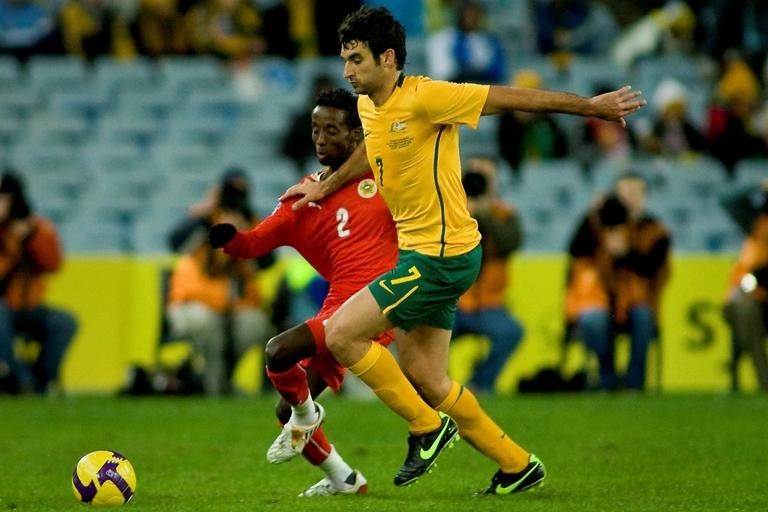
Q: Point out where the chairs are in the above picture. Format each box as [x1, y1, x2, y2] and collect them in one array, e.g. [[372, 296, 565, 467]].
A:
[[0, 26, 767, 397]]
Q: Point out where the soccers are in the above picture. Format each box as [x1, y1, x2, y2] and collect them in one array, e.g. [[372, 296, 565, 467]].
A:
[[72, 451, 137, 505]]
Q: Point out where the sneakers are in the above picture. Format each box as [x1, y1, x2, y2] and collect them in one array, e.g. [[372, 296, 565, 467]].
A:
[[474, 454, 547, 497], [264, 402, 327, 463], [297, 468, 368, 497], [393, 411, 461, 488]]
